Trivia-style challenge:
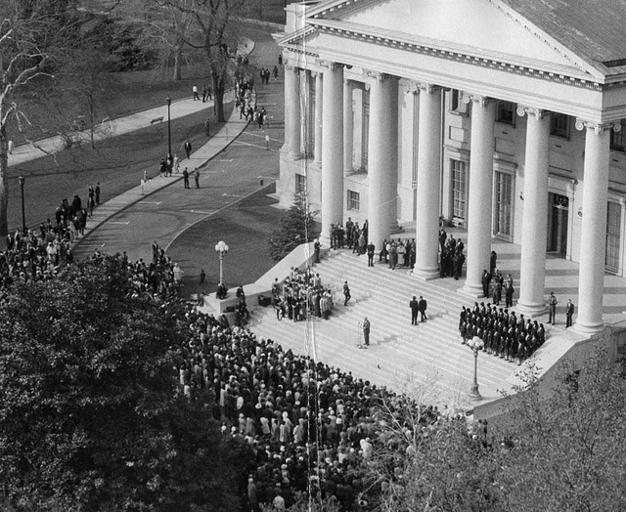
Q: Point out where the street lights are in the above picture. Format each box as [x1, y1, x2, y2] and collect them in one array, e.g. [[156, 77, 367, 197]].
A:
[[214, 240, 229, 288], [18, 174, 25, 237], [467, 335, 485, 401], [165, 96, 171, 154]]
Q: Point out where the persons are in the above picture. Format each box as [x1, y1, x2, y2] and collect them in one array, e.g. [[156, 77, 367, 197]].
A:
[[183, 167, 191, 190], [184, 140, 193, 160], [159, 153, 180, 178], [214, 264, 352, 328], [545, 291, 558, 326], [0, 180, 208, 303], [409, 296, 418, 324], [156, 303, 490, 512], [190, 53, 283, 136], [314, 237, 320, 264], [458, 303, 546, 366], [481, 249, 515, 307], [194, 168, 200, 189], [327, 217, 465, 280], [419, 294, 429, 322], [565, 298, 574, 328]]
